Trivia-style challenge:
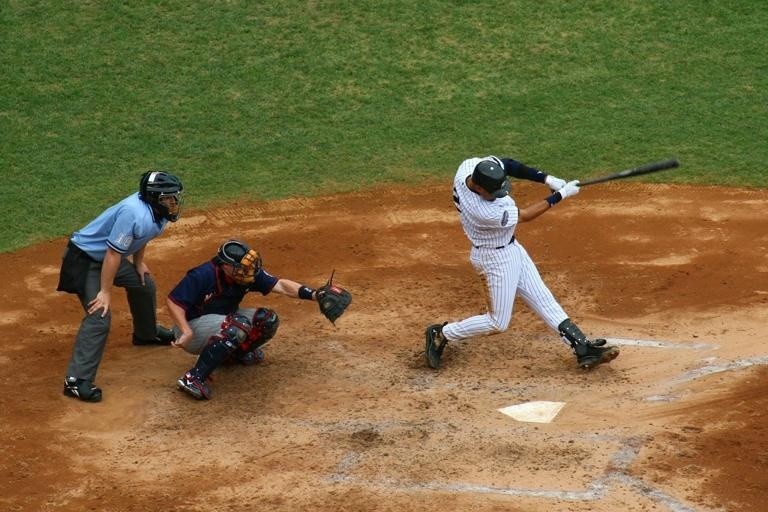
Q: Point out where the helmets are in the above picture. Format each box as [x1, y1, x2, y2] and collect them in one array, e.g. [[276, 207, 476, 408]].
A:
[[470, 160, 511, 198], [140, 170, 185, 222], [216, 239, 262, 287]]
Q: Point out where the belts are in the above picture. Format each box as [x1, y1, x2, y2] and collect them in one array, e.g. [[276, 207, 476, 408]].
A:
[[475, 235, 513, 249]]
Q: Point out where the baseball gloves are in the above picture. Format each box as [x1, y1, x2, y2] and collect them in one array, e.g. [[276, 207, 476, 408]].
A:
[[315, 285, 353, 322]]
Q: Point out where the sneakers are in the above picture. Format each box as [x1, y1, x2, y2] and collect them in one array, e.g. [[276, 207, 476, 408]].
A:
[[132, 326, 175, 346], [63, 376, 101, 402], [574, 345, 619, 368], [176, 376, 203, 400], [223, 351, 255, 368], [423, 321, 447, 370]]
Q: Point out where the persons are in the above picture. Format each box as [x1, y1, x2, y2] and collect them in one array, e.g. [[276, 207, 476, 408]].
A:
[[56, 169, 183, 403], [163, 238, 352, 399], [426, 155, 622, 371]]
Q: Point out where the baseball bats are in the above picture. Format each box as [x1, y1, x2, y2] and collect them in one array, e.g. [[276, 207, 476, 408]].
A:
[[576, 160, 679, 187]]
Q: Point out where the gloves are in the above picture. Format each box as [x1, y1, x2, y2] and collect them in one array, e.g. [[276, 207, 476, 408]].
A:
[[557, 180, 579, 200], [544, 174, 566, 191]]
